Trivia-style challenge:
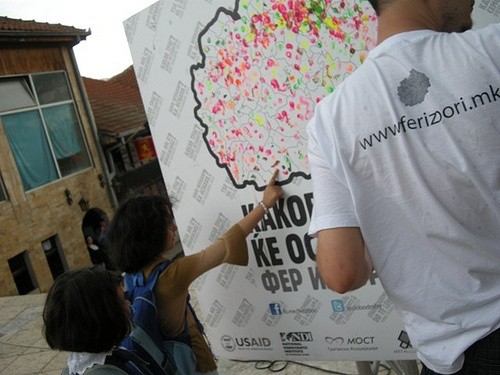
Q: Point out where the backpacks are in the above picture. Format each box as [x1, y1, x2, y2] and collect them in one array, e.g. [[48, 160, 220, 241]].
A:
[[106, 260, 200, 375]]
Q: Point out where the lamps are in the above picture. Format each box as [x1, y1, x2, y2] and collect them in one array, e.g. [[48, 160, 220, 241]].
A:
[[78, 196, 89, 212]]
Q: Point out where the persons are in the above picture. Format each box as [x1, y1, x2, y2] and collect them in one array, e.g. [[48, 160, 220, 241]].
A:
[[105, 170, 285, 375], [305, 0, 500, 375], [86, 235, 105, 270], [43, 266, 153, 375], [96, 218, 108, 251]]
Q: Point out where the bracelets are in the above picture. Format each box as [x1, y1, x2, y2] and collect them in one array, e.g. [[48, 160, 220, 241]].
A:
[[260, 201, 268, 214]]
